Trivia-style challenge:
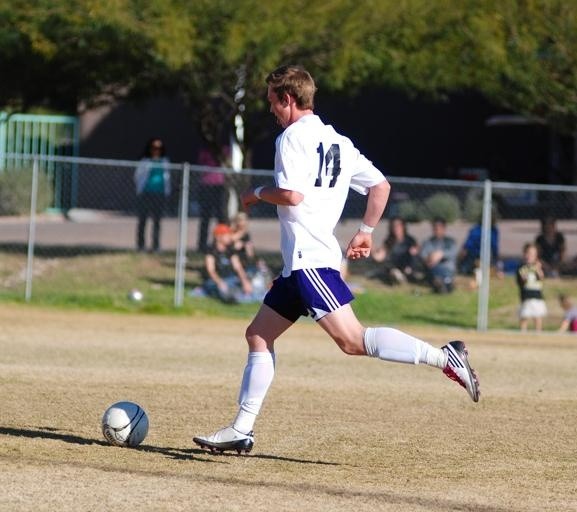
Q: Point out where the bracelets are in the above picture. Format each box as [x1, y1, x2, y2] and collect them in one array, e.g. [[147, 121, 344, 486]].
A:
[[358, 222, 375, 233], [253, 185, 264, 201]]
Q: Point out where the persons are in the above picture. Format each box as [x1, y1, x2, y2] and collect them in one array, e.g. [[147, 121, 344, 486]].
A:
[[193, 66, 480, 456], [133, 137, 172, 251], [372, 214, 577, 332], [229, 212, 255, 260], [192, 127, 229, 252], [203, 223, 267, 305]]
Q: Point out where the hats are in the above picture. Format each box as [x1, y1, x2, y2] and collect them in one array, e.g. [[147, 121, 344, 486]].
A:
[[213, 223, 236, 235]]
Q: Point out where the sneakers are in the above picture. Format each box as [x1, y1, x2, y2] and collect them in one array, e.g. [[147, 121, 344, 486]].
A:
[[192, 426, 254, 454], [441, 341, 481, 401]]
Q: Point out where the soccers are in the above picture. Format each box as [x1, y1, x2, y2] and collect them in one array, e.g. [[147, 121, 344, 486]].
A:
[[102, 401, 148, 447], [130, 290, 142, 300]]
